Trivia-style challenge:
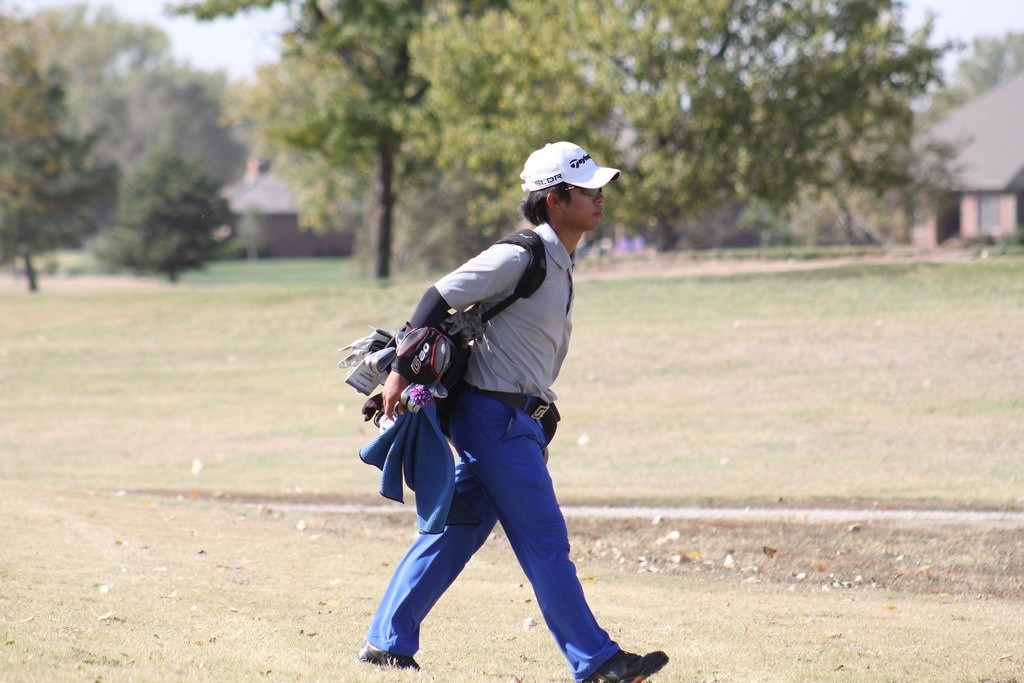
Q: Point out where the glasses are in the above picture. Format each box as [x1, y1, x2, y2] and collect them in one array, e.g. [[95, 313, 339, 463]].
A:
[[564, 183, 604, 196]]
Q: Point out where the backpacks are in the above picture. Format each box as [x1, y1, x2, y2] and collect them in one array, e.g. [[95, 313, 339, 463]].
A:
[[383, 229, 547, 404]]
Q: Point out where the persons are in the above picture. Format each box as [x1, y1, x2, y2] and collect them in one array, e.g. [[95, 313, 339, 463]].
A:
[[357, 141, 668, 683]]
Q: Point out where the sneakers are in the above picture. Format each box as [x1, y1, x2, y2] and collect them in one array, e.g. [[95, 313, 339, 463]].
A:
[[356, 642, 421, 674], [580, 646, 670, 683]]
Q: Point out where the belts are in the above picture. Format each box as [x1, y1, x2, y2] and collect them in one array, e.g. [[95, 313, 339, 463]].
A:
[[465, 383, 549, 421]]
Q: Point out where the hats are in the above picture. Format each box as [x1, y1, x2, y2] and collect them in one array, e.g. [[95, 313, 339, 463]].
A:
[[519, 141, 623, 188]]
